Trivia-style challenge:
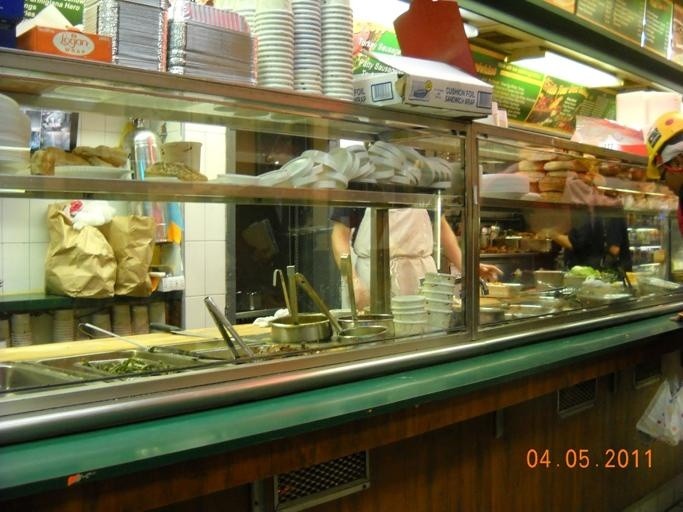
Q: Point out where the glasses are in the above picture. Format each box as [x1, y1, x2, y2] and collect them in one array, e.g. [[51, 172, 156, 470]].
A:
[[658, 168, 668, 186]]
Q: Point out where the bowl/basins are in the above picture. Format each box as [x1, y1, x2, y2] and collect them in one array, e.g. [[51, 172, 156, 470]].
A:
[[10, 311, 31, 347], [132, 306, 148, 335], [395, 320, 423, 337], [52, 308, 72, 343], [423, 281, 455, 292], [427, 309, 452, 325], [112, 304, 130, 336], [424, 289, 453, 300], [425, 297, 452, 310], [391, 305, 424, 311], [392, 295, 423, 305], [426, 271, 456, 283], [162, 141, 202, 171], [392, 312, 425, 321]]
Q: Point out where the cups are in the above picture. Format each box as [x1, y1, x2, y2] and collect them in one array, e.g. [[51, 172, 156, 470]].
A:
[[323, 0, 354, 102], [253, 1, 294, 92], [505, 238, 521, 249], [232, 0, 257, 87], [291, 0, 321, 96]]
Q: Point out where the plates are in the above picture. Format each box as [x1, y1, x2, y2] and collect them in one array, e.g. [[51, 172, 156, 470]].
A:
[[481, 172, 530, 197], [52, 165, 134, 181]]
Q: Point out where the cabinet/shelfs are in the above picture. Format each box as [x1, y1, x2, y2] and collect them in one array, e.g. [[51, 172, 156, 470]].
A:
[[0, 47, 683, 415]]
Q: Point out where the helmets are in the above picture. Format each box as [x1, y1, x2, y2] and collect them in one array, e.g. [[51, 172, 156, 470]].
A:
[[645, 110, 683, 180]]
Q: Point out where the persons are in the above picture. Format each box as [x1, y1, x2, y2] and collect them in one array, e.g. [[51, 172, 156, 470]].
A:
[[645, 113, 682, 235], [238, 205, 277, 310], [537, 212, 631, 282], [331, 206, 505, 326]]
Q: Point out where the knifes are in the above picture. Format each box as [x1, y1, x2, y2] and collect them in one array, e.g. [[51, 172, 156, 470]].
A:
[[152, 321, 210, 338]]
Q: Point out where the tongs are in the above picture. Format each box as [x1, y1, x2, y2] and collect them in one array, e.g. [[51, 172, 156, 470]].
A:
[[203, 297, 260, 362]]
